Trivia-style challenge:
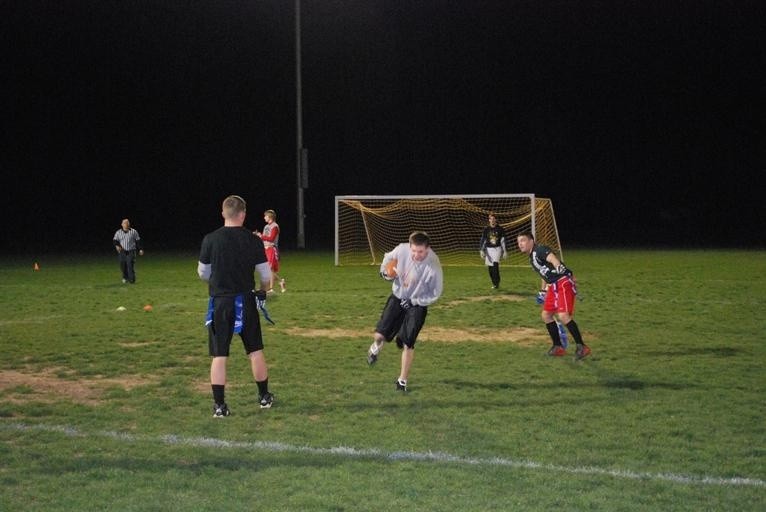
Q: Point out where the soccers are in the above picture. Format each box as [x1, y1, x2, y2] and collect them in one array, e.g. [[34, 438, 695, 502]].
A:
[[386, 260, 397, 277]]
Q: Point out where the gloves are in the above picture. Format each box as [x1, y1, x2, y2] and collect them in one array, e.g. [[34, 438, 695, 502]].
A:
[[536, 289, 546, 304], [479, 249, 486, 259], [399, 298, 414, 311], [503, 252, 508, 259]]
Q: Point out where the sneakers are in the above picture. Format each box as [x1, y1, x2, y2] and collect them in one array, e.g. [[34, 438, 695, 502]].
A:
[[491, 284, 498, 290], [366, 342, 384, 366], [212, 403, 230, 418], [547, 344, 566, 357], [122, 278, 135, 284], [574, 344, 591, 361], [396, 377, 408, 393], [266, 279, 287, 294], [258, 393, 275, 409]]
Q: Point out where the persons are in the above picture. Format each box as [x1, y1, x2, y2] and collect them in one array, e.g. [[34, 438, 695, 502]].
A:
[[480, 214, 508, 289], [254, 209, 286, 294], [112, 218, 144, 283], [367, 231, 443, 394], [517, 231, 591, 362], [197, 195, 275, 419]]
